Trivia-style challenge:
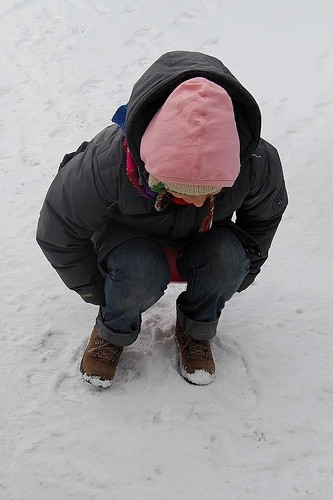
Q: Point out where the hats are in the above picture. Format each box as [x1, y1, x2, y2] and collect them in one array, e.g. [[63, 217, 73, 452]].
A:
[[147, 176, 229, 233]]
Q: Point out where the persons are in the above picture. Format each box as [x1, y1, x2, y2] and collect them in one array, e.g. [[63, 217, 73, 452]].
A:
[[36, 48, 290, 390]]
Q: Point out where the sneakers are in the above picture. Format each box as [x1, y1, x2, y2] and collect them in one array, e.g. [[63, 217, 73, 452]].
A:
[[80, 327, 123, 388], [175, 328, 215, 386]]
